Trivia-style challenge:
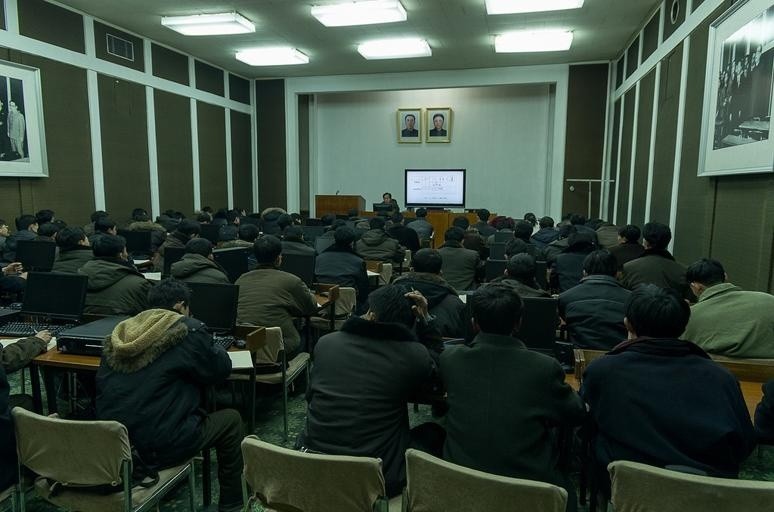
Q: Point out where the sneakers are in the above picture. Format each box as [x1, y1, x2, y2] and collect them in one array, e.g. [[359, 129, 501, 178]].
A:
[[218, 489, 254, 512]]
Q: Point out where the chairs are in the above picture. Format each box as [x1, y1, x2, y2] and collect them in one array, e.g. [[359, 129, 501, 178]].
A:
[[239, 434, 406, 509], [0, 194, 762, 460], [607, 460, 774, 509], [10, 406, 196, 509], [407, 445, 566, 508]]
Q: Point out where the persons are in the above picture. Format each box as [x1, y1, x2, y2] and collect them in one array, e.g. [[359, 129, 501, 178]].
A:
[[713, 44, 764, 149], [428, 112, 448, 138], [4, 100, 26, 159], [401, 112, 419, 138], [1, 202, 772, 511], [0, 101, 8, 157], [378, 192, 400, 211]]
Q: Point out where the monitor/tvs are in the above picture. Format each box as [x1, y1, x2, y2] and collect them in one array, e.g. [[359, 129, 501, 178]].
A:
[[464, 294, 559, 355], [405, 169, 466, 210], [14, 204, 548, 290], [20, 271, 88, 317], [183, 282, 240, 334]]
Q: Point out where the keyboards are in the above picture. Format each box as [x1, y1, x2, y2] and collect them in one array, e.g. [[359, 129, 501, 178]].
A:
[[0, 322, 78, 337], [212, 338, 235, 350]]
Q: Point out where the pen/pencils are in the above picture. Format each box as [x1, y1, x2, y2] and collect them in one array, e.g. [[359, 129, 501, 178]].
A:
[[30, 325, 38, 336], [411, 287, 429, 327]]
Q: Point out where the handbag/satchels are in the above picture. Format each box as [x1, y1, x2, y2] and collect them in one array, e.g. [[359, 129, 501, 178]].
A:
[[45, 445, 159, 498]]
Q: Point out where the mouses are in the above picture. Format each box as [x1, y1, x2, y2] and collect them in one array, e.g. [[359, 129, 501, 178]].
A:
[[319, 292, 329, 297], [561, 364, 574, 374], [234, 340, 246, 349]]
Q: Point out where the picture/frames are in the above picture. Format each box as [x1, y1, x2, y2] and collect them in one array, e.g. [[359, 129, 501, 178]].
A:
[[0, 58, 50, 181], [397, 108, 421, 144], [425, 107, 451, 143], [697, 1, 774, 177]]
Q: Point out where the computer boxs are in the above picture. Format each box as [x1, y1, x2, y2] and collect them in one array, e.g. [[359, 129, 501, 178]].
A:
[[0, 308, 22, 324], [56, 317, 134, 356]]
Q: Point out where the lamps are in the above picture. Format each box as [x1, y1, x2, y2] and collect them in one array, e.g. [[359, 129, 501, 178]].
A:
[[159, 0, 586, 67]]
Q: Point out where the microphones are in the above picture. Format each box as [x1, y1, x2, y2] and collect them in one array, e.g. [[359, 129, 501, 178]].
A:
[[336, 191, 339, 194]]
